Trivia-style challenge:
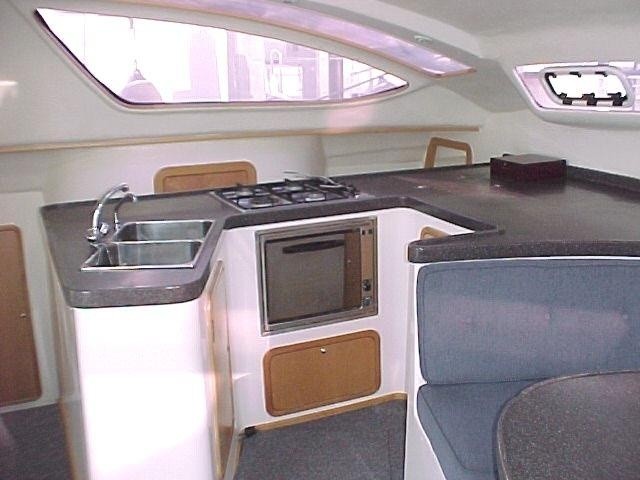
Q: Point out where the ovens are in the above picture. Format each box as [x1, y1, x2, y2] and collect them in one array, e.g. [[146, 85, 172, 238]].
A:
[[257, 217, 379, 335]]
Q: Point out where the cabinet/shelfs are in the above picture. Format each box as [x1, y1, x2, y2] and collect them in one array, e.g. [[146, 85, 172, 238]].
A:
[[76, 271, 237, 480], [0, 225, 43, 408]]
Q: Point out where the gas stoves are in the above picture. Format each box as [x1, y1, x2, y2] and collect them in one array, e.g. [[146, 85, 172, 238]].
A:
[[210, 174, 370, 214]]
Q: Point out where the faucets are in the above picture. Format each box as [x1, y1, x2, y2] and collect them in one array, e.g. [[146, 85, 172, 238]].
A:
[[86, 182, 129, 242]]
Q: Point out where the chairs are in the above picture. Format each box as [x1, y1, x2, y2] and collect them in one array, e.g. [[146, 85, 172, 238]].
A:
[[397, 258, 640, 480]]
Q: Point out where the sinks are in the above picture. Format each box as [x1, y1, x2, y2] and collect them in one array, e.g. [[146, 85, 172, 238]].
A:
[[112, 218, 216, 244], [79, 240, 204, 269]]
